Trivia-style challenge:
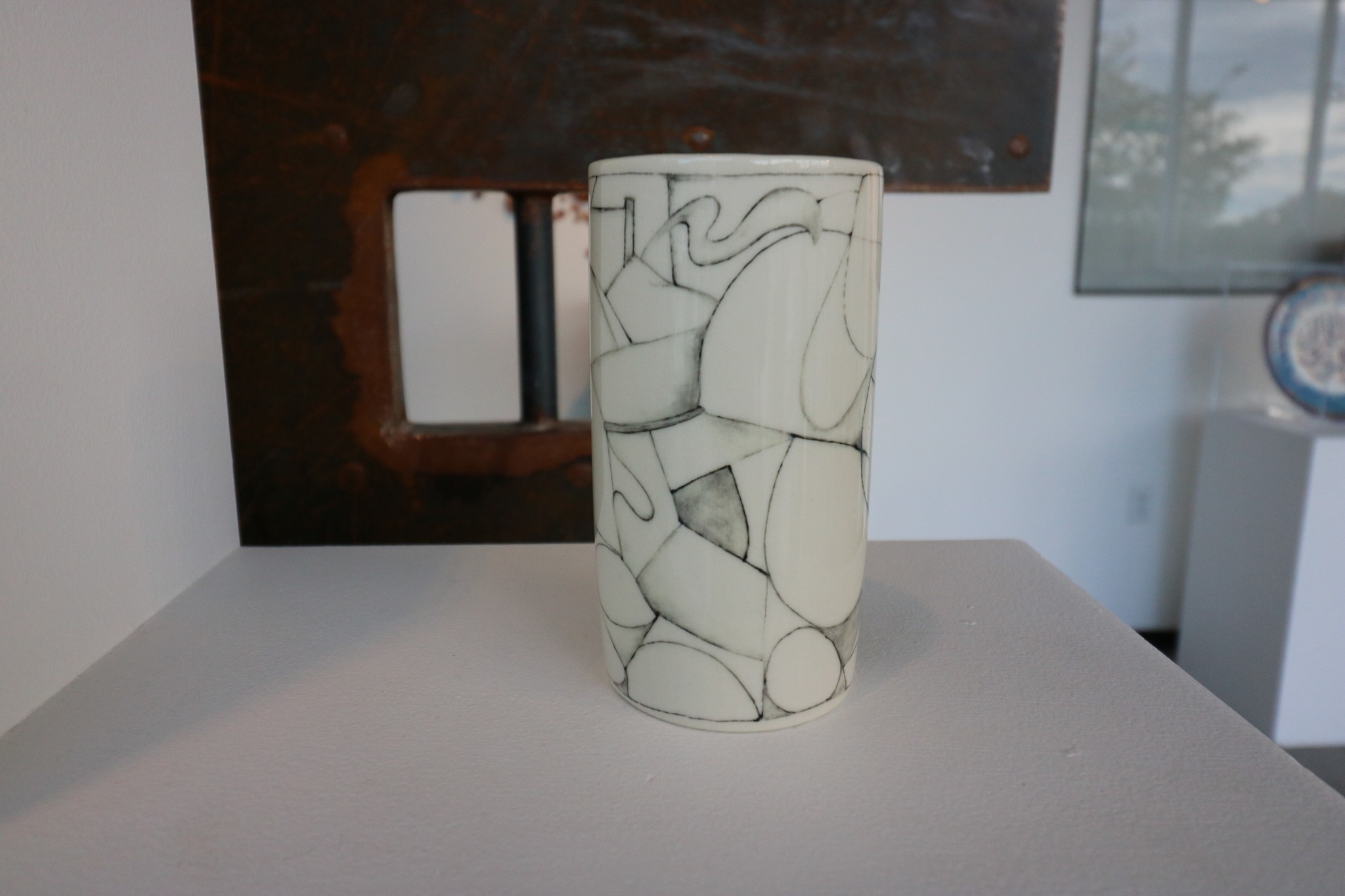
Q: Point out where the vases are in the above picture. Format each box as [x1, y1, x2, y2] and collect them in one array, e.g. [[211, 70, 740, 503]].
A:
[[585, 153, 881, 729]]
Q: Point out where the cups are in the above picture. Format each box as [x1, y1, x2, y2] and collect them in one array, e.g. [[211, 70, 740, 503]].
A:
[[584, 147, 886, 739]]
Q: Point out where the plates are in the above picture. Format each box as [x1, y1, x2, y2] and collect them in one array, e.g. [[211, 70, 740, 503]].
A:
[[1263, 274, 1345, 422]]
